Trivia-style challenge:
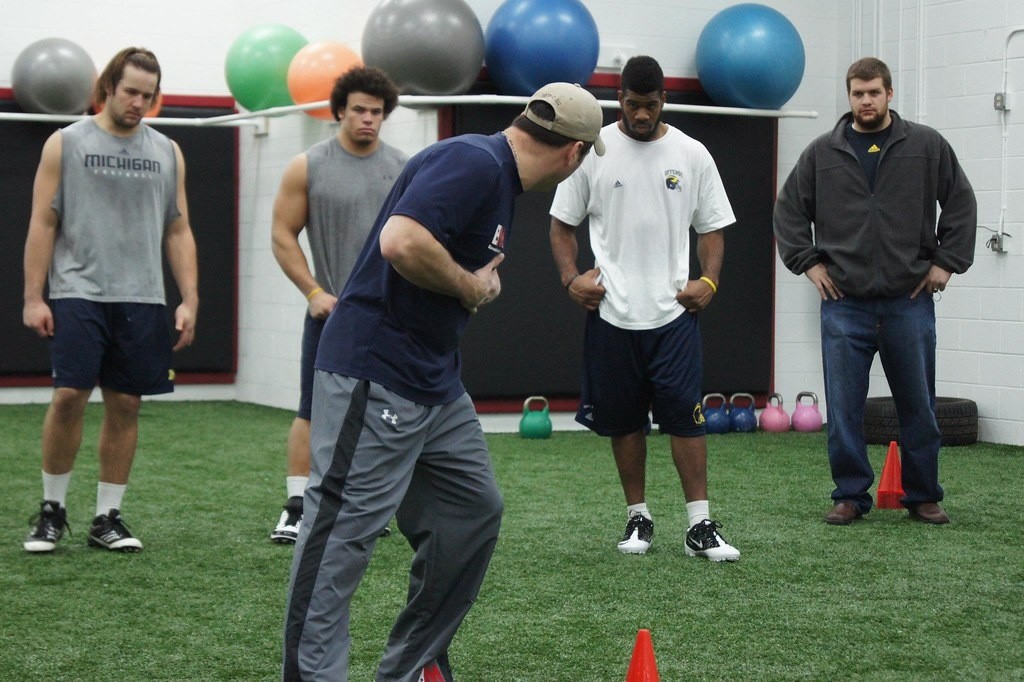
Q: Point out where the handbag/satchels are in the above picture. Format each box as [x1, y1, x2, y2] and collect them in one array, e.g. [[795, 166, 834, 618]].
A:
[[877, 442, 907, 510]]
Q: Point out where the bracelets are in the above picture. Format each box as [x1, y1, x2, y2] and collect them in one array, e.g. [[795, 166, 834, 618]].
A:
[[699, 276, 716, 293], [565, 273, 579, 290], [307, 287, 323, 302]]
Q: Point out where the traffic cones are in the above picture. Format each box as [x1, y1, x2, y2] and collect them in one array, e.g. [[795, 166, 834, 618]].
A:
[[626, 629, 660, 682], [876, 441, 907, 509]]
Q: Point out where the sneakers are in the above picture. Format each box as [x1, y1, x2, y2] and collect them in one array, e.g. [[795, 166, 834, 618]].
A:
[[23, 500, 67, 551], [618, 513, 654, 553], [684, 520, 742, 561], [88, 507, 145, 553], [270, 496, 304, 543]]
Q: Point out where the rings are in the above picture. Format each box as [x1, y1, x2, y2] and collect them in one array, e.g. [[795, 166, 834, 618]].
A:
[[933, 288, 937, 292]]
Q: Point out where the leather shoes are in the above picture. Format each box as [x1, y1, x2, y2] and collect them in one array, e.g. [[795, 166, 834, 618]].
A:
[[907, 503, 950, 523], [824, 501, 864, 524]]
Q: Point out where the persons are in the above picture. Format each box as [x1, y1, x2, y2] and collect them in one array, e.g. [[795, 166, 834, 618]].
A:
[[271, 65, 410, 546], [22, 46, 198, 552], [284, 82, 606, 682], [550, 54, 742, 562], [773, 57, 977, 525]]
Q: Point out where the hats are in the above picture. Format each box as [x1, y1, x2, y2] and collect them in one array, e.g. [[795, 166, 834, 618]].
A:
[[521, 81, 606, 155]]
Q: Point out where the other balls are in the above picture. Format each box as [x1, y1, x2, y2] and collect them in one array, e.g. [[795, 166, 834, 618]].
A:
[[11, 0, 809, 122]]
